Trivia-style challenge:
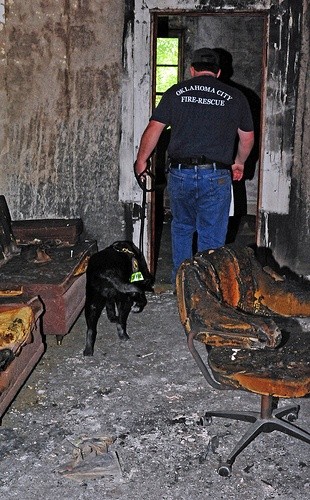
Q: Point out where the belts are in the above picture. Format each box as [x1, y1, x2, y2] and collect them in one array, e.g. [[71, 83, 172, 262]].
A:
[[170, 161, 227, 170]]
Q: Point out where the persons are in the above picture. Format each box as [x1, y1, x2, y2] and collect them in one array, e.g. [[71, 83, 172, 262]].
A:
[[134, 48, 254, 296]]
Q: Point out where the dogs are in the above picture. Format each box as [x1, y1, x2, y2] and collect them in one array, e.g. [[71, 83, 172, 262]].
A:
[[83, 241, 154, 356]]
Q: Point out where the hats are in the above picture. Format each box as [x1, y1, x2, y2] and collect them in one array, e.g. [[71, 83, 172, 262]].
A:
[[191, 48, 220, 65]]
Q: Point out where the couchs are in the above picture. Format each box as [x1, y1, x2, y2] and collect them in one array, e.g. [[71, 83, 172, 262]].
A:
[[0, 291, 45, 419], [0, 195, 98, 346]]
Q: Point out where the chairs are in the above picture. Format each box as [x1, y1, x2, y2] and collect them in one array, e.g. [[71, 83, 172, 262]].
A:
[[174, 243, 310, 477]]
